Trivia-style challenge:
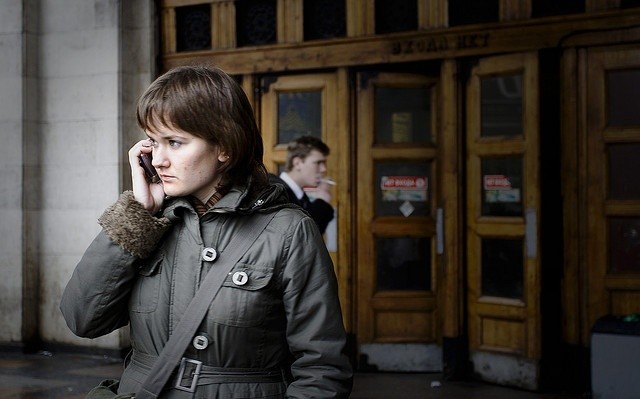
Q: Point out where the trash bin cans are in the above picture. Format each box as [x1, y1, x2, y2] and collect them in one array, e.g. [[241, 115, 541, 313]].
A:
[[590, 314, 640, 399]]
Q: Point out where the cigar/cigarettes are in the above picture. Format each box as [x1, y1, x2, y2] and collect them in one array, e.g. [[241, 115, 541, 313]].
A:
[[319, 178, 337, 186]]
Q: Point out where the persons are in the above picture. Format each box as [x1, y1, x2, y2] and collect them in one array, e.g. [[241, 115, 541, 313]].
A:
[[278, 135, 335, 241], [58, 64, 354, 399]]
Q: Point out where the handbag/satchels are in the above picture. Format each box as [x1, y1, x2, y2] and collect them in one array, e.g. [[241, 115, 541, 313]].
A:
[[86, 377, 135, 399]]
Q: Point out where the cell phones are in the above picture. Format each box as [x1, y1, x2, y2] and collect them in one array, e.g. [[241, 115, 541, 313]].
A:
[[139, 152, 162, 184]]
